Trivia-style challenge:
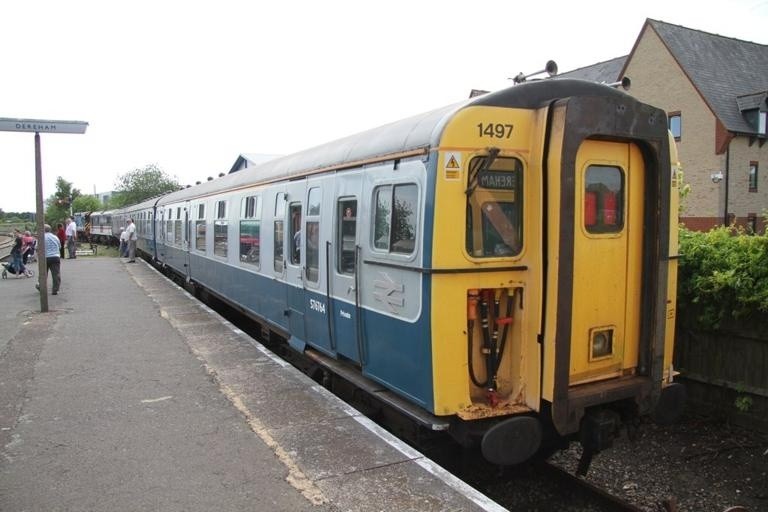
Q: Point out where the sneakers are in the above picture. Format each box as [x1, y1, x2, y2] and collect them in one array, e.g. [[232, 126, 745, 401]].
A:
[[51, 289, 59, 295], [35, 283, 41, 292]]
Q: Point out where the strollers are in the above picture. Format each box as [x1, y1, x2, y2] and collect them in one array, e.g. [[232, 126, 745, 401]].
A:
[[0, 237, 36, 280]]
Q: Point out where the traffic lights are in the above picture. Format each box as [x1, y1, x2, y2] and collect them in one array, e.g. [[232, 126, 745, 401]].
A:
[[56, 197, 63, 205]]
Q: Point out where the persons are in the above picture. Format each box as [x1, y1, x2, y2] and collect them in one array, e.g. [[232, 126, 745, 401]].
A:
[[10, 216, 138, 295], [294, 230, 301, 263]]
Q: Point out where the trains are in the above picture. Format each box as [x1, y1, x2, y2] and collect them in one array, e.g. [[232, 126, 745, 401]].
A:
[[73, 60, 684, 478]]
